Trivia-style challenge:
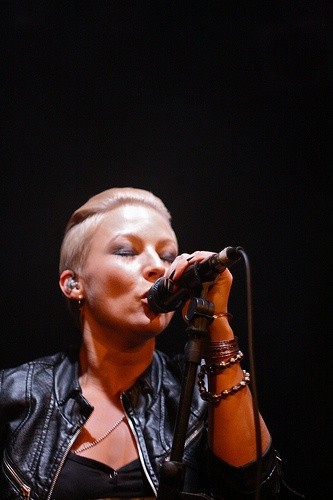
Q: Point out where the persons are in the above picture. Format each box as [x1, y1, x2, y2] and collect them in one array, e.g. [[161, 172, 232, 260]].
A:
[[0, 188, 287, 500]]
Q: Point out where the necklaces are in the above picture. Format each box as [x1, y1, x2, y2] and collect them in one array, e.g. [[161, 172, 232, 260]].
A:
[[70, 414, 127, 455]]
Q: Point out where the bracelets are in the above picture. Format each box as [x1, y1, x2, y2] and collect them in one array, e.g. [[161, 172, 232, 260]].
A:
[[202, 337, 239, 362], [208, 312, 233, 323], [198, 351, 250, 407]]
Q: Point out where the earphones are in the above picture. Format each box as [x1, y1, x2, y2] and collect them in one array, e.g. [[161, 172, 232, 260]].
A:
[[70, 282, 78, 290]]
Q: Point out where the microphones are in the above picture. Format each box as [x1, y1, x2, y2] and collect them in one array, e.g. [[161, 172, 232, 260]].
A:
[[147, 246, 241, 313]]
